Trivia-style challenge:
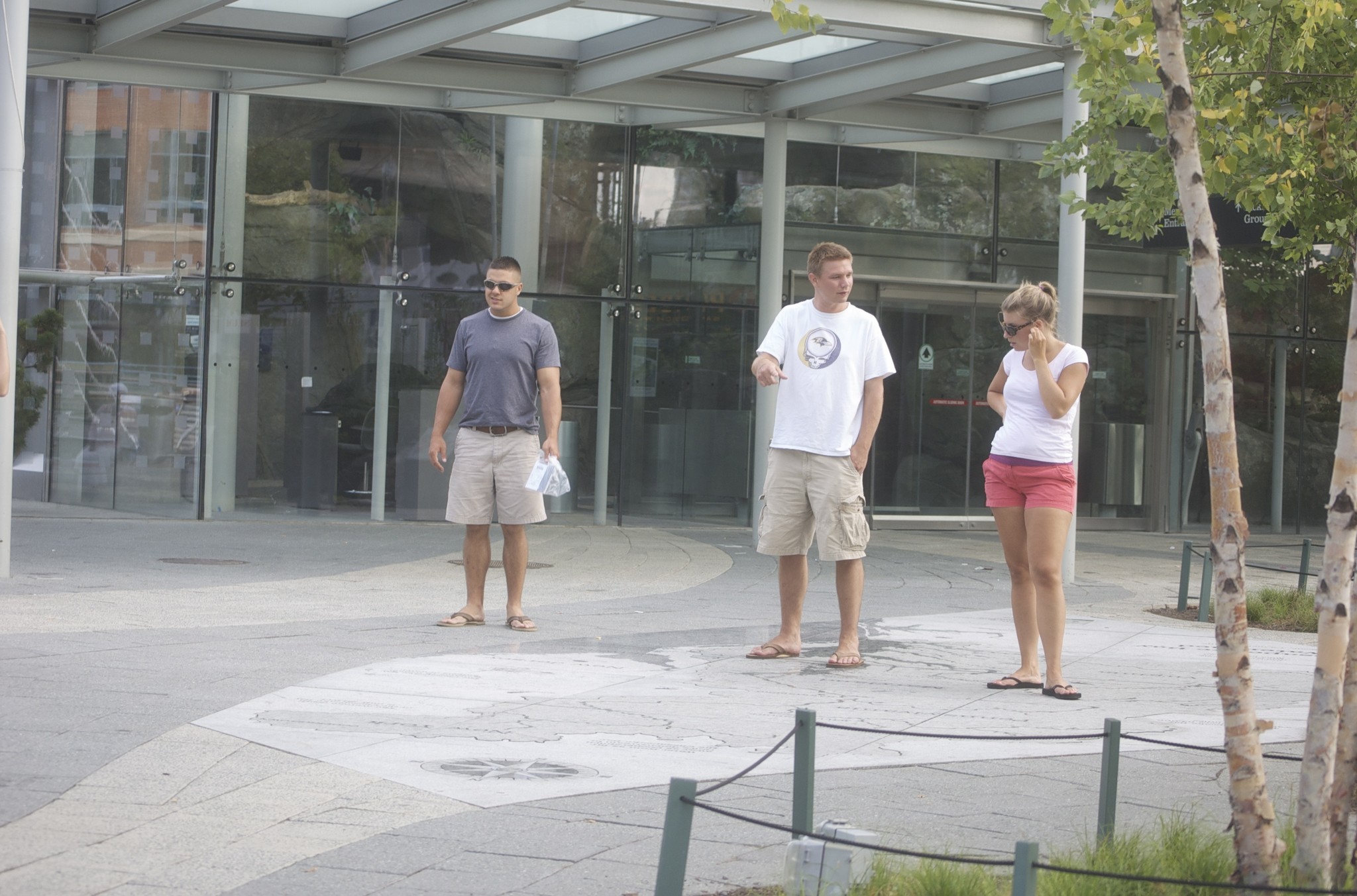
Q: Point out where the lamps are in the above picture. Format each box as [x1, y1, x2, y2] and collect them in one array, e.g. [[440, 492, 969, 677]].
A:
[[394, 271, 411, 307], [738, 248, 758, 259], [173, 260, 235, 296], [339, 140, 364, 162], [782, 293, 787, 302], [979, 247, 1008, 258], [1178, 317, 1185, 349], [1293, 325, 1316, 355], [607, 283, 643, 320]]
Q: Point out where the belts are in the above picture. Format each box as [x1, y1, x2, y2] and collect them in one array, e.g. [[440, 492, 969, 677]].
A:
[[468, 426, 518, 436]]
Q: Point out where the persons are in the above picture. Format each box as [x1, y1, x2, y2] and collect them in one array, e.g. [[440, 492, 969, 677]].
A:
[[751, 242, 896, 669], [429, 256, 562, 631], [982, 282, 1089, 699]]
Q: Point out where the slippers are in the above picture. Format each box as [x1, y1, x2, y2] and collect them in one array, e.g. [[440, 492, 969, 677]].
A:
[[505, 616, 538, 631], [826, 651, 864, 669], [437, 613, 486, 627], [746, 642, 800, 658], [987, 677, 1044, 689], [1042, 685, 1081, 699]]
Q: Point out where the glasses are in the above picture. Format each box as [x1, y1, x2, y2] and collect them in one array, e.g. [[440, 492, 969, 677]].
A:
[[1000, 317, 1038, 335], [483, 279, 519, 291]]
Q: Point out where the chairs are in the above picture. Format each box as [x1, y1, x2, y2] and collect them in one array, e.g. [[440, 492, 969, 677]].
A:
[[338, 406, 400, 505]]
